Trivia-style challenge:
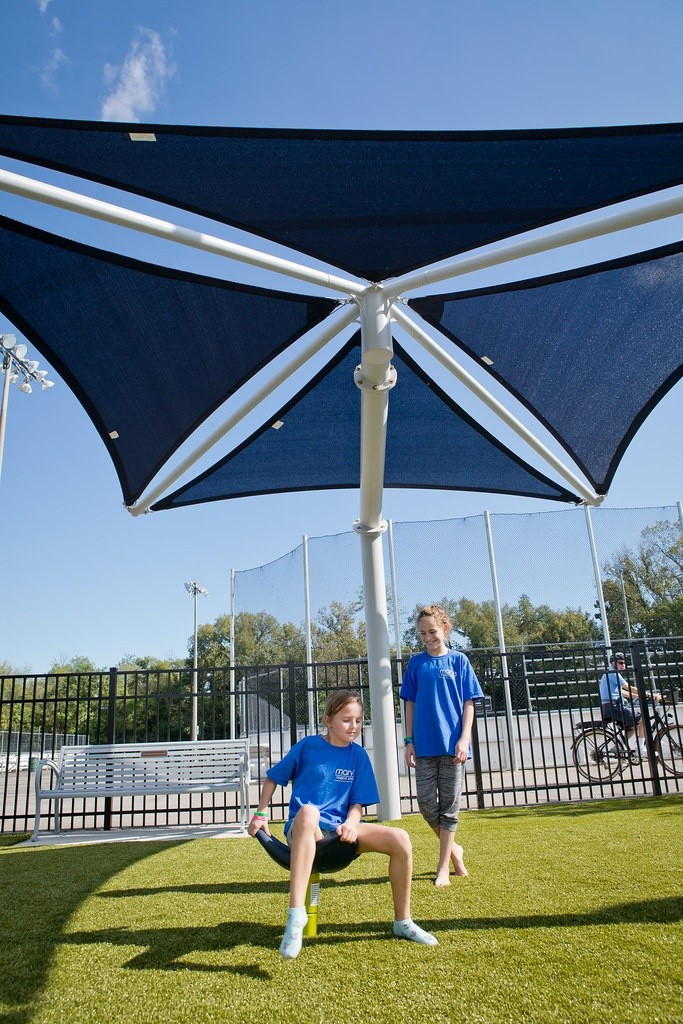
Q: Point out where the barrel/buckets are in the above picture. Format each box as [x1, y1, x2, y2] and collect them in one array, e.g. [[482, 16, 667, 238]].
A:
[[30, 757, 39, 772]]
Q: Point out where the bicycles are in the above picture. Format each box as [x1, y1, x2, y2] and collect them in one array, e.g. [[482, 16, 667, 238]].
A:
[[570, 685, 683, 783]]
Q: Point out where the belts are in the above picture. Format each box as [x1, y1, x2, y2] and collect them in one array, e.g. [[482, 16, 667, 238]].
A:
[[603, 703, 611, 708]]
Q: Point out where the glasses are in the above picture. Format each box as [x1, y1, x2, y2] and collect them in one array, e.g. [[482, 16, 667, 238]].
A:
[[614, 661, 625, 664]]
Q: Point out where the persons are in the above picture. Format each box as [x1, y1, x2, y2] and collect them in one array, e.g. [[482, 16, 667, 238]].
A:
[[398, 605, 484, 887], [599, 653, 662, 763], [248, 690, 437, 957]]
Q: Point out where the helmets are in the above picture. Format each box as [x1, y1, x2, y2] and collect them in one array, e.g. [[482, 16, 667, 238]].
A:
[[610, 652, 625, 663]]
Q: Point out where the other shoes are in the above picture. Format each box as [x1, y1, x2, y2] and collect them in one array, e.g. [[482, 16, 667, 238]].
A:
[[635, 747, 660, 758], [611, 746, 631, 764]]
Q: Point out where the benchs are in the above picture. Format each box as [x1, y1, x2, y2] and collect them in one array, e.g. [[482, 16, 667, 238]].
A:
[[249, 745, 269, 769], [30, 739, 250, 842]]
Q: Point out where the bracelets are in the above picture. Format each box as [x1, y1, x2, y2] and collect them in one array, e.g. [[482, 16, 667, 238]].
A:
[[403, 736, 413, 746], [253, 815, 268, 821], [254, 812, 268, 817]]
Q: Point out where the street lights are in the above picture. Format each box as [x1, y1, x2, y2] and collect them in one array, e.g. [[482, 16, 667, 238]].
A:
[[0, 332, 55, 474], [184, 582, 208, 785]]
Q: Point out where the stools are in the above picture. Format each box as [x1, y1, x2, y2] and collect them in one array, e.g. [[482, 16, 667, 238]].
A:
[[256, 828, 358, 938]]
